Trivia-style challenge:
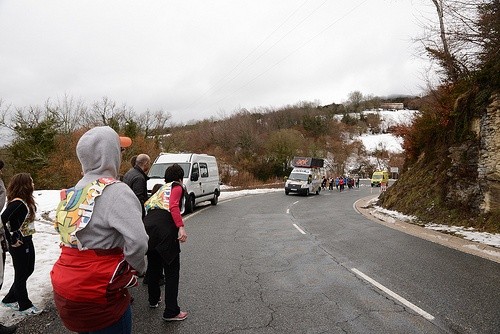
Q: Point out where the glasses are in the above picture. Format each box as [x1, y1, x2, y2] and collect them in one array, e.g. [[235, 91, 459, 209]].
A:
[[121, 148, 125, 153]]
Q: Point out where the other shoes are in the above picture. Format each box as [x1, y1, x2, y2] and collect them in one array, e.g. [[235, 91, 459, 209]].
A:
[[162, 311, 188, 320], [0, 322, 16, 334], [17, 307, 43, 315], [149, 298, 162, 307], [1, 302, 19, 307]]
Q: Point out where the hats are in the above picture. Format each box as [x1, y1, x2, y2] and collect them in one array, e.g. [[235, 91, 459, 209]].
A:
[[118, 134, 131, 148]]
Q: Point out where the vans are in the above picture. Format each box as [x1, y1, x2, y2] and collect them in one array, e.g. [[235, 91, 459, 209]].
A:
[[146, 152, 221, 213], [371, 171, 389, 187], [284, 157, 325, 196]]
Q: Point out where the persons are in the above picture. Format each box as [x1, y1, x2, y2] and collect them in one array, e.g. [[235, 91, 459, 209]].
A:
[[0, 160, 7, 290], [321, 176, 328, 192], [50, 125, 149, 334], [335, 176, 360, 192], [122, 155, 152, 218], [328, 177, 334, 191], [144, 163, 189, 321], [1, 173, 44, 315]]
